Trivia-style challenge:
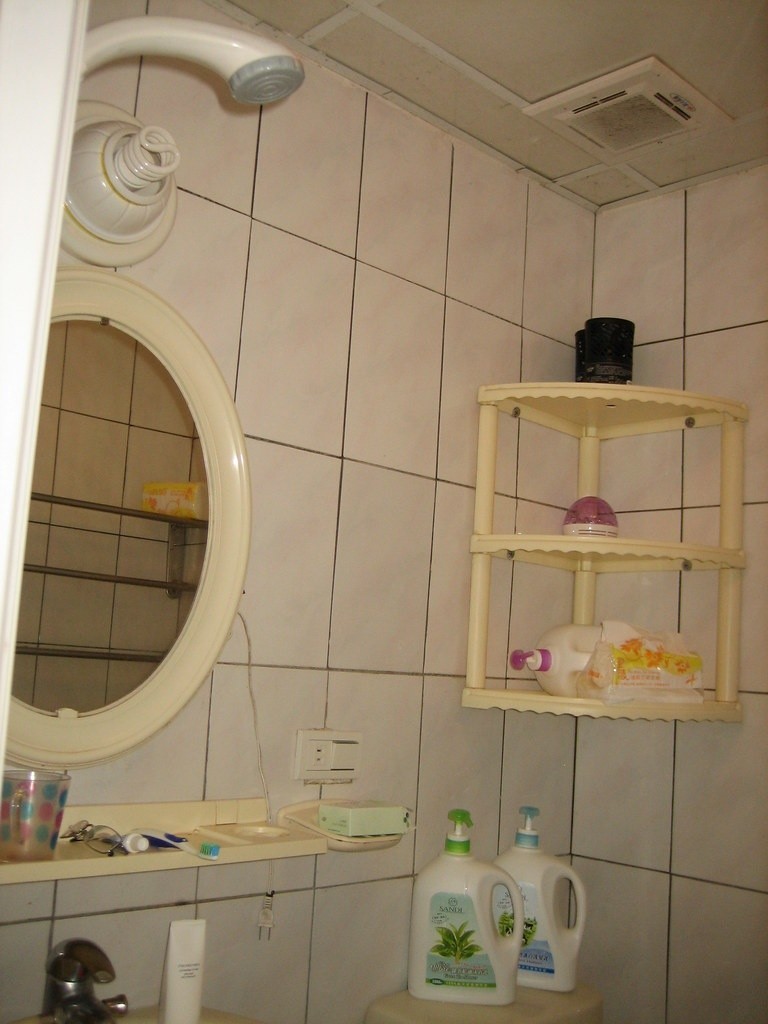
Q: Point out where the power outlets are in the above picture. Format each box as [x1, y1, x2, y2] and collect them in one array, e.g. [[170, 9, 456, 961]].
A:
[[291, 728, 364, 785]]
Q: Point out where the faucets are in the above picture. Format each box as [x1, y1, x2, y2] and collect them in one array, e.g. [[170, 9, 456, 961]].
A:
[[37, 941, 128, 1024]]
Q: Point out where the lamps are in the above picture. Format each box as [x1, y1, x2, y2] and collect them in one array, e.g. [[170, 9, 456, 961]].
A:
[[62, 98, 186, 268]]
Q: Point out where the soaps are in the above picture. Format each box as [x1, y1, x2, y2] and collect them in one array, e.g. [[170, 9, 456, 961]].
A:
[[317, 799, 419, 841]]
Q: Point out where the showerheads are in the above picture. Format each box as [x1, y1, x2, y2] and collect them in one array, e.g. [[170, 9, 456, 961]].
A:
[[81, 10, 311, 109]]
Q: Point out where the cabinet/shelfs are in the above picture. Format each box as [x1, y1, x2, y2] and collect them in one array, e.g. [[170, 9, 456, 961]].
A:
[[0, 798, 405, 889], [460, 381, 751, 728]]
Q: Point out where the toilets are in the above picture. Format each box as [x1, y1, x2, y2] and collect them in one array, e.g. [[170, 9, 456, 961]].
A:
[[365, 976, 604, 1024]]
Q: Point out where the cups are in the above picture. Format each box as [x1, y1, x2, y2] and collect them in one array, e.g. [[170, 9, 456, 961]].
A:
[[1, 770, 71, 863]]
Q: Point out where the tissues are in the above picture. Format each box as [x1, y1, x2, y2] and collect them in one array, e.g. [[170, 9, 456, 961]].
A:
[[140, 479, 210, 522], [574, 620, 709, 703]]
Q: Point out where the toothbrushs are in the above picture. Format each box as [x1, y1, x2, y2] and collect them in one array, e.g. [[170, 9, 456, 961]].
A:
[[129, 825, 222, 863]]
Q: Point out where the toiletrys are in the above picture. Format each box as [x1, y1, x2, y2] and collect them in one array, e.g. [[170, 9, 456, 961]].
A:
[[153, 917, 210, 1023], [486, 803, 593, 997], [408, 807, 527, 1009], [509, 615, 645, 697], [109, 832, 151, 856]]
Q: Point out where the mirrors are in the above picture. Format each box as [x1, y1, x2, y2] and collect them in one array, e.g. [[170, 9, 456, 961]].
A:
[[9, 315, 209, 717]]
